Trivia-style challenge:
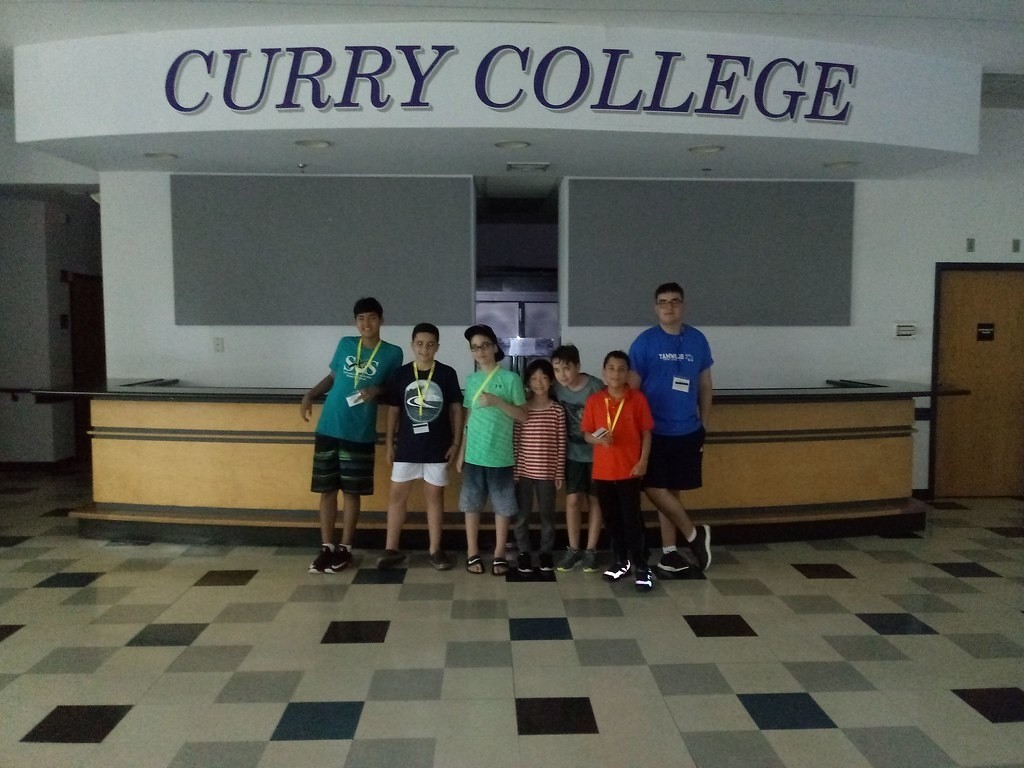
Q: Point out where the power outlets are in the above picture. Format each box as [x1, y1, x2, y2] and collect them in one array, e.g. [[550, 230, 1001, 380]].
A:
[[211, 337, 224, 352]]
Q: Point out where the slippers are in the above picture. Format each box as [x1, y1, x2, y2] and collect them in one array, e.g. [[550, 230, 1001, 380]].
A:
[[467, 555, 484, 573], [492, 557, 509, 575]]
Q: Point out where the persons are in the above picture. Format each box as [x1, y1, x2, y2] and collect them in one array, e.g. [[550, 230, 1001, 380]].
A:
[[582, 352, 655, 591], [548, 346, 642, 572], [375, 323, 462, 570], [511, 360, 567, 572], [627, 282, 712, 574], [456, 324, 528, 575], [299, 297, 402, 573]]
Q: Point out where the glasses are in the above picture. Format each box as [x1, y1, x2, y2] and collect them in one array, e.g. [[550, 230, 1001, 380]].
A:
[[470, 343, 493, 352], [657, 299, 683, 308]]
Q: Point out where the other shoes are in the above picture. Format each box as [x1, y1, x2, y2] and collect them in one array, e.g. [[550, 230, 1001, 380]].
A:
[[376, 549, 406, 569], [427, 551, 451, 569]]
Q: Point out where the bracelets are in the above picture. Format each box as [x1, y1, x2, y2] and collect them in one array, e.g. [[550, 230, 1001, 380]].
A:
[[451, 444, 460, 448]]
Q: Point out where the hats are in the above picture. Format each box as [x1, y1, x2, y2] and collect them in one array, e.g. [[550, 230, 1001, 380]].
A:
[[464, 324, 504, 362]]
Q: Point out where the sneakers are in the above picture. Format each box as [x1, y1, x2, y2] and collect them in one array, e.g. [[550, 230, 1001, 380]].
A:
[[517, 552, 533, 572], [324, 546, 352, 573], [557, 546, 582, 572], [635, 567, 652, 587], [309, 546, 334, 574], [539, 551, 554, 571], [583, 549, 600, 572], [687, 525, 711, 570], [656, 551, 689, 571], [603, 560, 631, 580]]
